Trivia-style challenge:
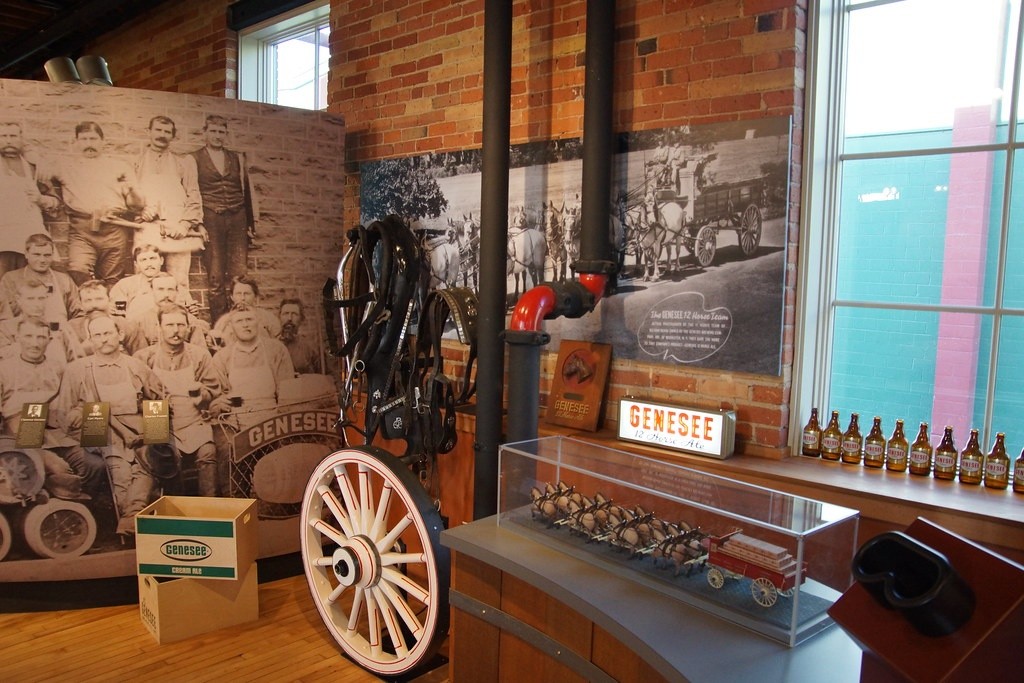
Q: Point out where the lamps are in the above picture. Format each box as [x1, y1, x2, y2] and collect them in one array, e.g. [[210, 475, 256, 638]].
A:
[[76, 53, 113, 85], [41, 56, 81, 84]]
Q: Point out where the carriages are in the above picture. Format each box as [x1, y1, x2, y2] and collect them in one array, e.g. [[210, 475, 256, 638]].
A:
[[608, 151, 771, 283]]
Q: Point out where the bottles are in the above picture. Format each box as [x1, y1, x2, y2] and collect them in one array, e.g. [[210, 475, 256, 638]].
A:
[[932, 425, 958, 481], [958, 428, 985, 485], [842, 413, 863, 465], [1012, 446, 1024, 494], [984, 429, 1011, 490], [885, 418, 909, 472], [908, 421, 933, 476], [863, 416, 887, 468], [821, 410, 843, 460], [802, 407, 822, 457]]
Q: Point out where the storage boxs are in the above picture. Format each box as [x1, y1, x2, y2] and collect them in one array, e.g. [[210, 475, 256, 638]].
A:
[[133, 495, 257, 580], [139, 565, 258, 641]]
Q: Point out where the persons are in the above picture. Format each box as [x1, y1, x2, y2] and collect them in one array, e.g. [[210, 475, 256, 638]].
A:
[[277, 298, 329, 376], [0, 234, 87, 360], [0, 119, 61, 282], [24, 122, 147, 282], [124, 271, 209, 355], [105, 243, 212, 351], [57, 312, 172, 540], [667, 141, 686, 185], [650, 137, 670, 187], [131, 301, 223, 498], [129, 115, 203, 305], [0, 317, 107, 505], [210, 301, 297, 500], [0, 279, 83, 372], [211, 274, 284, 345], [62, 279, 151, 363], [181, 114, 260, 324]]
